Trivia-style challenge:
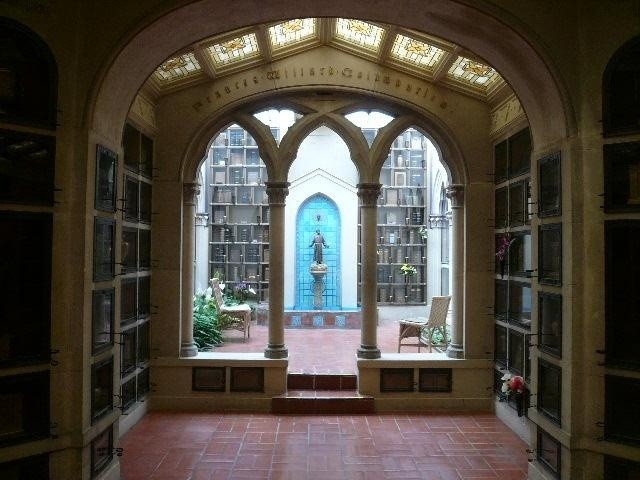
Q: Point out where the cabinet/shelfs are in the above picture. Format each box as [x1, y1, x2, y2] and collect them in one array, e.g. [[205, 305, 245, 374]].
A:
[[357, 128, 429, 306], [206, 126, 279, 305]]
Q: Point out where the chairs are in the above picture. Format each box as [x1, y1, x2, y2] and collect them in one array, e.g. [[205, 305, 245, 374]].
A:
[[397, 294, 452, 352], [209, 278, 252, 342]]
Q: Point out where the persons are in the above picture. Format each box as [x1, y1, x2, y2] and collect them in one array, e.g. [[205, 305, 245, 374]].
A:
[[309, 229, 326, 264]]
